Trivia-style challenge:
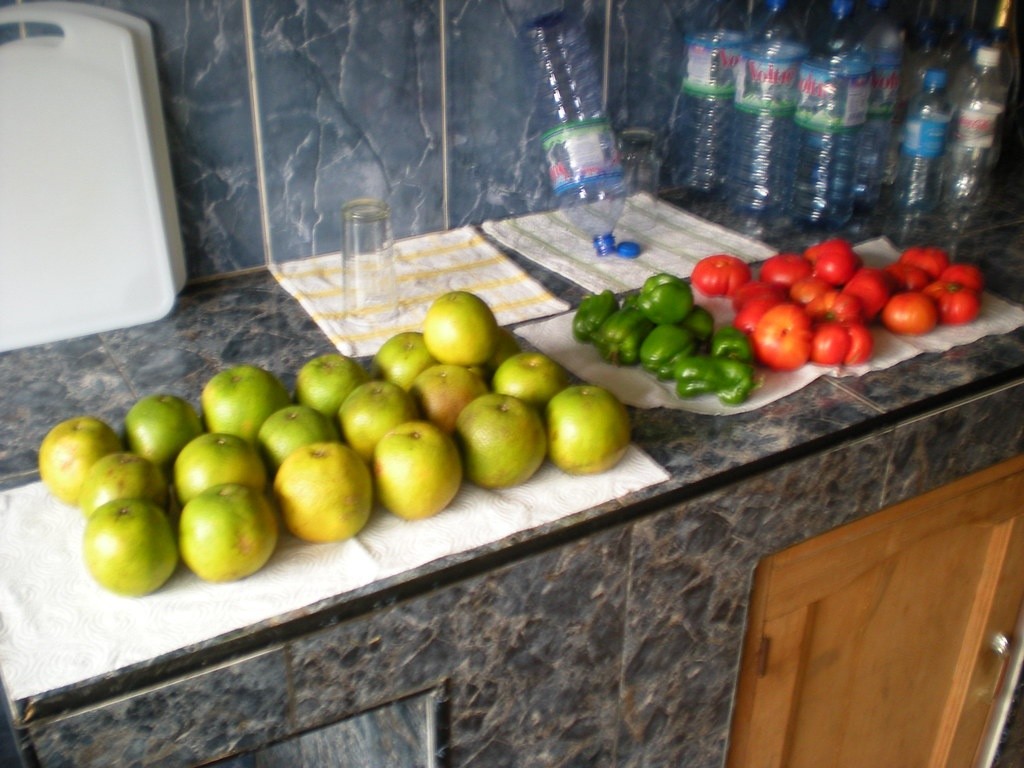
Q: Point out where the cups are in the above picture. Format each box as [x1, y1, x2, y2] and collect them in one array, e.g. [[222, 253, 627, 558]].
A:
[[342, 198, 400, 325]]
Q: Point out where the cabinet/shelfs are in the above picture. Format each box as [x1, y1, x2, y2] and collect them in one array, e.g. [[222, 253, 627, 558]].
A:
[[733, 452, 1024, 768]]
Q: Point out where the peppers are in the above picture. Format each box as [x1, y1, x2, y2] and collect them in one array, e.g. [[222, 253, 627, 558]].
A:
[[573, 274, 767, 405]]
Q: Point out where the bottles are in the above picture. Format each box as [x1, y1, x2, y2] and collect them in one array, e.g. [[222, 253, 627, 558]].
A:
[[664, 0, 1016, 235], [515, 13, 640, 260]]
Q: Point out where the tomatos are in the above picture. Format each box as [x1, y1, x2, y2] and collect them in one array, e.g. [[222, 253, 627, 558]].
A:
[[693, 239, 985, 371]]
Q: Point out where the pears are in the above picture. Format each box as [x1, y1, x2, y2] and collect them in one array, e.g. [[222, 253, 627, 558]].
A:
[[39, 290, 628, 597]]
